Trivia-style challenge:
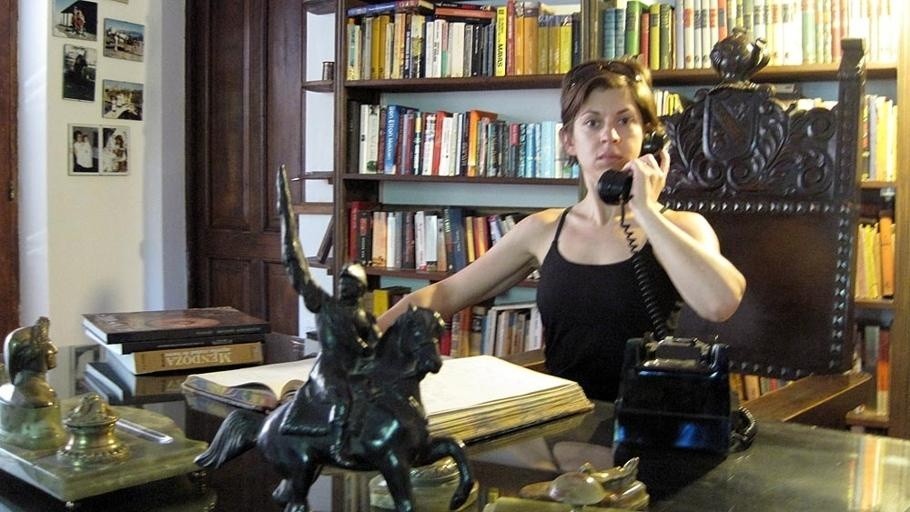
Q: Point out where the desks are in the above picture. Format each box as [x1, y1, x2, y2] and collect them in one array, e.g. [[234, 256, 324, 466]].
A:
[[0, 332, 910, 512]]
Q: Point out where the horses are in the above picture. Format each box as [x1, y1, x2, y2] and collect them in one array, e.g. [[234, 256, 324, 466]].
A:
[[192, 303, 475, 512]]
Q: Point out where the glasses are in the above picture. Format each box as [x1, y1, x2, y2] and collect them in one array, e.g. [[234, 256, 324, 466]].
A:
[[566, 58, 645, 91]]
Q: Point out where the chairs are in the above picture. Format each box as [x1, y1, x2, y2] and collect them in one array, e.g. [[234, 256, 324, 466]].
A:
[[498, 29, 876, 430]]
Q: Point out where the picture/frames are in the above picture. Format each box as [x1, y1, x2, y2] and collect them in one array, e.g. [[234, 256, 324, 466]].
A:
[[51, 0, 99, 42], [68, 123, 100, 176], [63, 43, 98, 103], [103, 18, 145, 62], [99, 125, 131, 175], [102, 79, 144, 121]]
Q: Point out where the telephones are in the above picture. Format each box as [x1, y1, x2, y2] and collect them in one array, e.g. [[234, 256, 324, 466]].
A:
[[597, 130, 664, 206]]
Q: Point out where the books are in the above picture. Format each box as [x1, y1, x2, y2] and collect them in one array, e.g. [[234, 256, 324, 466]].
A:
[[345, 98, 579, 179], [169, 344, 595, 453], [599, 0, 899, 72], [855, 212, 896, 305], [653, 87, 690, 115], [350, 200, 540, 279], [732, 325, 888, 414], [343, 1, 580, 81], [361, 289, 542, 358], [789, 95, 899, 182], [72, 306, 273, 407]]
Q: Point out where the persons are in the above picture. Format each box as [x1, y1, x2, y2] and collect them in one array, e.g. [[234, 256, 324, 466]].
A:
[[379, 58, 746, 405], [104, 134, 128, 172], [274, 164, 378, 476], [81, 135, 91, 156], [73, 131, 94, 172]]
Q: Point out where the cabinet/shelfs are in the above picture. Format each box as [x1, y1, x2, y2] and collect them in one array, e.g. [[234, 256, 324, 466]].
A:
[[292, 0, 910, 440]]
